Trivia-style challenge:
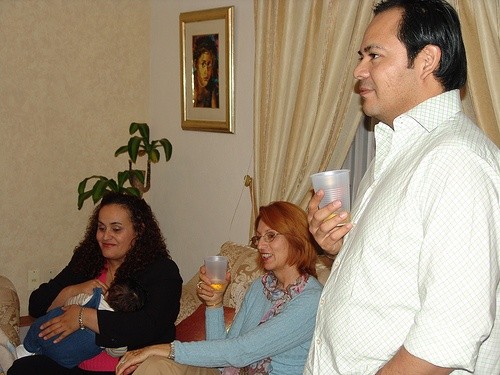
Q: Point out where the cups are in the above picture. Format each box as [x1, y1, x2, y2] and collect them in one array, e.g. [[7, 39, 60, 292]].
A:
[[310, 170, 350, 226], [204, 256, 227, 293]]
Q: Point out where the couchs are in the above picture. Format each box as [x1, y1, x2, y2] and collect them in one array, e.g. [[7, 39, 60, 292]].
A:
[[14, 240, 335, 375]]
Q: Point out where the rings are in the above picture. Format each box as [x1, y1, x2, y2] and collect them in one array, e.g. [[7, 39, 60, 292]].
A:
[[197, 280, 205, 289], [121, 362, 124, 364]]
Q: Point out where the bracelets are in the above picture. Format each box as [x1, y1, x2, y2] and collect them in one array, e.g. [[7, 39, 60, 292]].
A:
[[206, 300, 223, 307], [79, 307, 85, 330], [169, 342, 175, 359]]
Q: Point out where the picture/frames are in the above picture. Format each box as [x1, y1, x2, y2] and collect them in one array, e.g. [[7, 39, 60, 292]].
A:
[[179, 5, 235, 134]]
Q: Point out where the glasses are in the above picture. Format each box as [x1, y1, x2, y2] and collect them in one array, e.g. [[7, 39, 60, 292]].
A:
[[250, 230, 283, 246]]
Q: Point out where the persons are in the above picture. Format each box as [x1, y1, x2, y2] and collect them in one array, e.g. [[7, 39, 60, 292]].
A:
[[6, 194, 182, 375], [115, 201, 325, 375], [306, 0, 500, 375]]
